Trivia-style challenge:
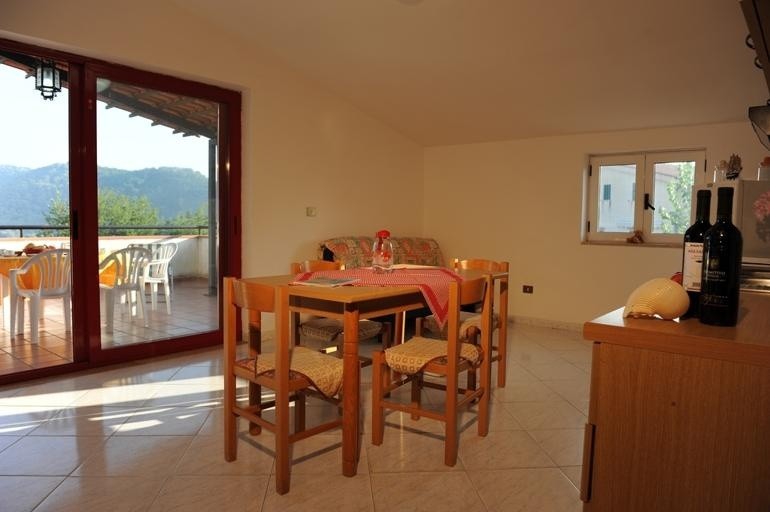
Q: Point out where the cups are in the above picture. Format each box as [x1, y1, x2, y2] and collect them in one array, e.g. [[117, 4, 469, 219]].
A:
[[15, 252, 22, 256], [461, 260, 468, 270], [5, 250, 12, 254]]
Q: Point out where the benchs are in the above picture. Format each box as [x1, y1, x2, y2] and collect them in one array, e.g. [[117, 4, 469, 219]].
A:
[[321, 236, 447, 345]]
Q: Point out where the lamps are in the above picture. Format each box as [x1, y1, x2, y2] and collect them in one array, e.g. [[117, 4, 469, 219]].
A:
[[36, 60, 62, 101]]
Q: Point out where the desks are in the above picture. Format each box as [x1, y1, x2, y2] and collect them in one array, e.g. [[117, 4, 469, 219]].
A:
[[240, 265, 507, 446], [578, 286, 770, 512], [1, 253, 125, 335]]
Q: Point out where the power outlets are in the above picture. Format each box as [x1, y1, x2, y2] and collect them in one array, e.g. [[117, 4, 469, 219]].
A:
[[523, 285, 533, 293]]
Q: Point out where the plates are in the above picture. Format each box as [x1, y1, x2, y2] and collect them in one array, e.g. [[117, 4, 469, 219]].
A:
[[27, 254, 38, 257], [4, 254, 18, 257]]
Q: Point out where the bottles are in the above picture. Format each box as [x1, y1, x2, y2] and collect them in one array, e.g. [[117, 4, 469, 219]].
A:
[[683, 190, 713, 316], [372, 229, 393, 274], [699, 187, 742, 328], [713, 165, 726, 184]]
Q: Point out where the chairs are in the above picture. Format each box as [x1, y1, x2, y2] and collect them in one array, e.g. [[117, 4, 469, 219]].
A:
[[372, 273, 493, 466], [223, 277, 373, 495], [9, 249, 70, 345], [290, 260, 391, 416], [124, 242, 178, 321], [415, 258, 509, 403], [98, 247, 154, 335]]
